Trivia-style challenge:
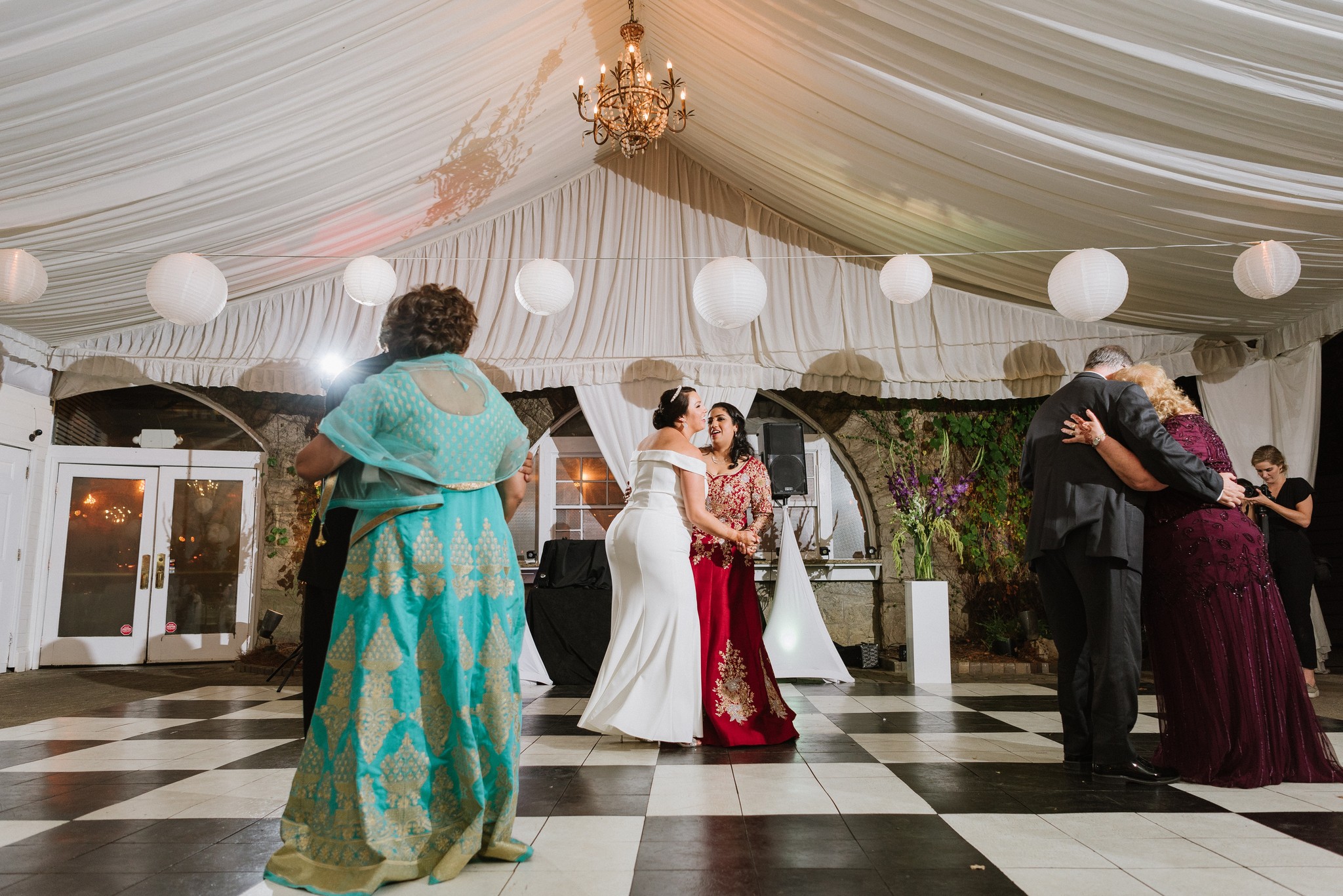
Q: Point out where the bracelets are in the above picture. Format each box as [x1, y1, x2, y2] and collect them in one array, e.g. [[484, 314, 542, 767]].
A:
[[744, 529, 753, 532]]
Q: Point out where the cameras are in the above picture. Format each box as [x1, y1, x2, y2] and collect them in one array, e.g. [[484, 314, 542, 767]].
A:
[[1242, 484, 1270, 498]]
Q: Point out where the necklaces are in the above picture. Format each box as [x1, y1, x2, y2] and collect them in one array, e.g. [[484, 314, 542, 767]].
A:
[[712, 452, 730, 464]]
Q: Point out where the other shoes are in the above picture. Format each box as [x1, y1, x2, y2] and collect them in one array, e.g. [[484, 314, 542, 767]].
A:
[[1306, 683, 1319, 698]]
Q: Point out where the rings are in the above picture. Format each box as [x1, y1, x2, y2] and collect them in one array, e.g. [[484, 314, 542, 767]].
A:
[[623, 496, 626, 500], [1070, 430, 1074, 435], [752, 546, 754, 550], [1071, 424, 1075, 429]]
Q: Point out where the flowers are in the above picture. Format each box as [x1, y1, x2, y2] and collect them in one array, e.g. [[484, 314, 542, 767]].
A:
[[876, 428, 984, 578]]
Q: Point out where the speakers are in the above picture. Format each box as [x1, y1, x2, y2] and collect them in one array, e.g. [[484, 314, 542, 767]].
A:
[[756, 423, 808, 500]]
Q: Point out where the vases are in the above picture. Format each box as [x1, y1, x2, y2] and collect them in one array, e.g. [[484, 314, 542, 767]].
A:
[[913, 538, 935, 581]]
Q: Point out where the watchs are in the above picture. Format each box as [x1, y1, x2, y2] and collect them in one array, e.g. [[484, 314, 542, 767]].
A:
[[1092, 433, 1108, 448]]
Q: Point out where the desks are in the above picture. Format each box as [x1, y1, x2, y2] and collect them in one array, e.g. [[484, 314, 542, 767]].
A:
[[904, 581, 951, 684]]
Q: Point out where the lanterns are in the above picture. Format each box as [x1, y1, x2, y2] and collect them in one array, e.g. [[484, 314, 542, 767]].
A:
[[0, 249, 49, 304], [343, 255, 397, 306], [1233, 239, 1301, 300], [693, 256, 767, 329], [1048, 247, 1129, 322], [514, 259, 574, 316], [879, 255, 933, 304], [146, 253, 228, 326]]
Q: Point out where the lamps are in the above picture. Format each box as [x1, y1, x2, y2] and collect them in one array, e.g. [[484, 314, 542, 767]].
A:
[[573, 0, 695, 159], [255, 609, 284, 646]]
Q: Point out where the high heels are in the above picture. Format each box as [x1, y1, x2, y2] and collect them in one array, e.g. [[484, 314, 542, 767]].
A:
[[657, 737, 702, 748], [620, 735, 654, 743]]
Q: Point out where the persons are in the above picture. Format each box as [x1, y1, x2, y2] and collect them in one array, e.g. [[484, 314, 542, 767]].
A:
[[1062, 361, 1343, 789], [577, 384, 760, 747], [623, 401, 800, 748], [1017, 345, 1247, 786], [1243, 445, 1319, 698], [263, 282, 535, 896], [299, 295, 533, 741]]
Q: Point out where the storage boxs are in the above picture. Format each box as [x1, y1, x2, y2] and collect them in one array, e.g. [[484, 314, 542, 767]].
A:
[[855, 642, 879, 668]]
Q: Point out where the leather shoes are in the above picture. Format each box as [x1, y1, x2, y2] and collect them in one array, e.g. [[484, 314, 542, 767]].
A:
[[1063, 753, 1092, 770], [1092, 753, 1180, 786]]
